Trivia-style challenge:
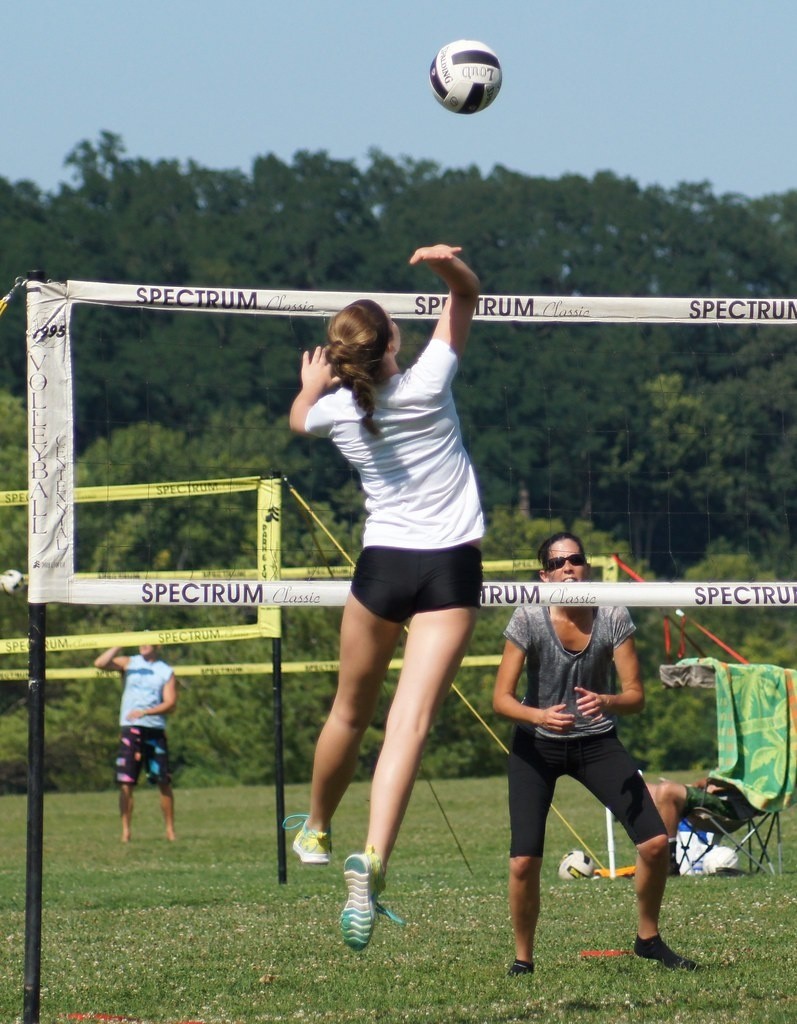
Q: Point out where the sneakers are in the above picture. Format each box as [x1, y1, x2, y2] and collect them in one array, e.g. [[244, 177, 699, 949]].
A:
[[292, 819, 331, 864], [339, 851, 385, 951]]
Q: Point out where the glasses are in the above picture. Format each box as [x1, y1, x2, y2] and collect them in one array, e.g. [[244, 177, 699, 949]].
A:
[[546, 554, 585, 571]]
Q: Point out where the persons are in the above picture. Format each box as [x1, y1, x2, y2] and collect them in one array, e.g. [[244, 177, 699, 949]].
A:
[[290, 244, 485, 950], [491, 530, 701, 980], [643, 769, 767, 879], [94, 638, 178, 846]]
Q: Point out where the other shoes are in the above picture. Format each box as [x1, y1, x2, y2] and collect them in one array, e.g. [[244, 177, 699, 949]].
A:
[[667, 864, 679, 875]]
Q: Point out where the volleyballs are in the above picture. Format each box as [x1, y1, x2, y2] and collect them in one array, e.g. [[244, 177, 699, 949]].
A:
[[427, 37, 505, 116], [556, 850, 596, 882]]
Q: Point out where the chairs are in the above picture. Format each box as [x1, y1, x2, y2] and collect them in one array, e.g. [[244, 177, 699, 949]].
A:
[[659, 656, 797, 875]]
